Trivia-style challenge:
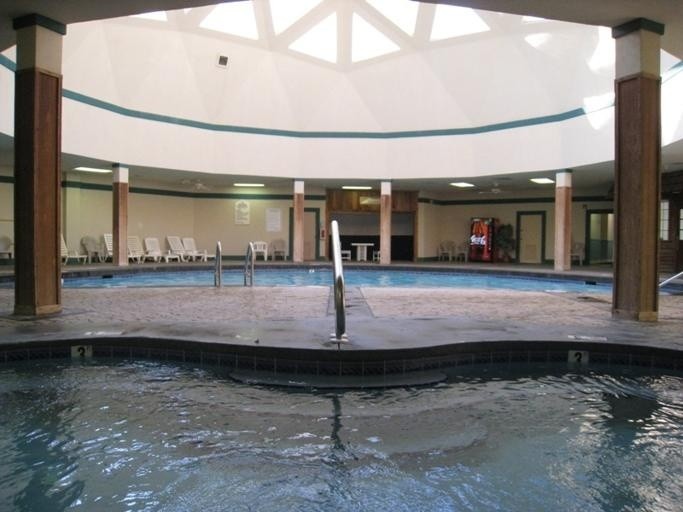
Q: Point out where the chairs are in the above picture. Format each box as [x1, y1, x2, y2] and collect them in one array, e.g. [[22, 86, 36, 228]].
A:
[[341, 241, 351, 262], [0, 236, 12, 259], [60, 233, 217, 265], [372, 250, 381, 262], [438, 242, 452, 262], [270, 239, 287, 261], [571, 240, 586, 266], [252, 241, 268, 262], [454, 241, 467, 263]]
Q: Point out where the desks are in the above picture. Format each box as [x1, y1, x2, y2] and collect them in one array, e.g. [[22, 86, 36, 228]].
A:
[[351, 243, 374, 262]]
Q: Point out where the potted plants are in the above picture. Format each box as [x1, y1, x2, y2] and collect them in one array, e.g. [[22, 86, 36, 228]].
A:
[[495, 224, 513, 263]]
